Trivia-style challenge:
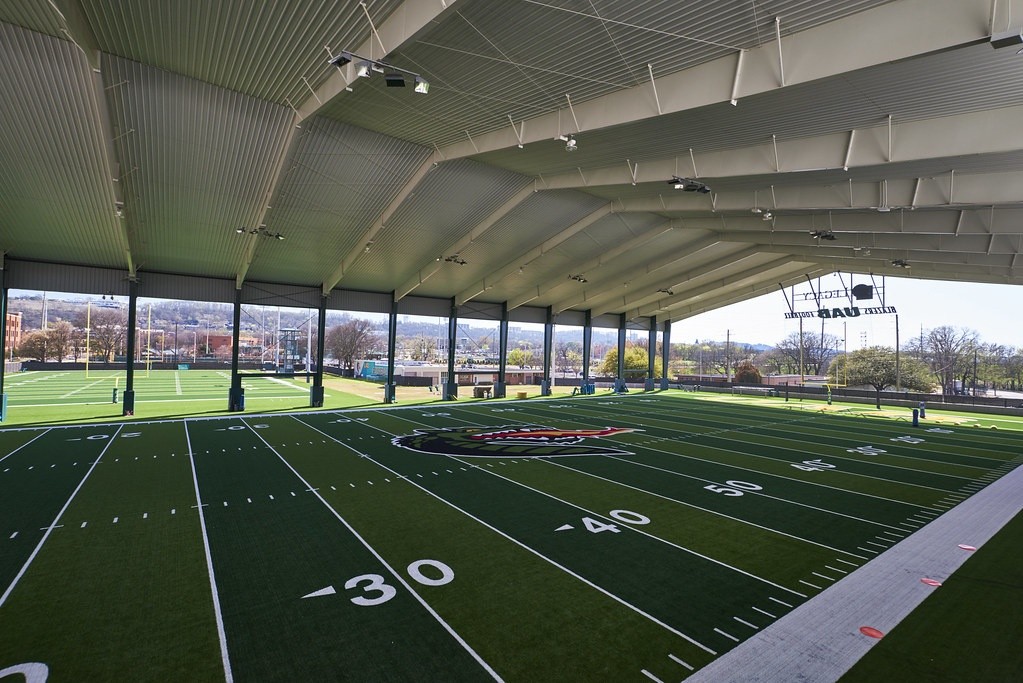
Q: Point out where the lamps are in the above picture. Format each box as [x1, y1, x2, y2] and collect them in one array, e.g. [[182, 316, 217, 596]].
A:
[[236, 225, 286, 241], [668, 176, 711, 195], [570, 275, 586, 284], [891, 260, 913, 271], [438, 254, 467, 265], [659, 288, 673, 297], [327, 50, 430, 96], [809, 230, 837, 242]]
